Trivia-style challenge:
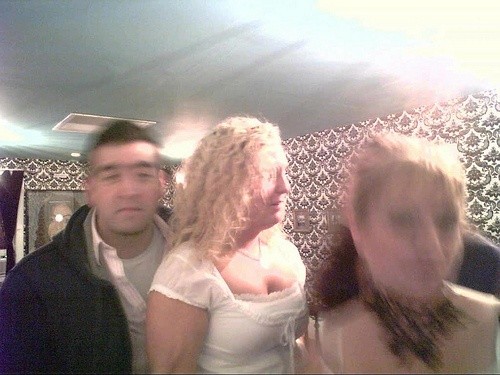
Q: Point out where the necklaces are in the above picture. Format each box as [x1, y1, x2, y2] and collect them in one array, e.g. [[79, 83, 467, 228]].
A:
[[236, 237, 270, 271]]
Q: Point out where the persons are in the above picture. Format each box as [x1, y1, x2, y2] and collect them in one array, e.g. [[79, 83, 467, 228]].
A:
[[34, 196, 81, 250], [146, 116, 310, 375], [301, 135, 500, 375], [0, 120, 176, 375]]
